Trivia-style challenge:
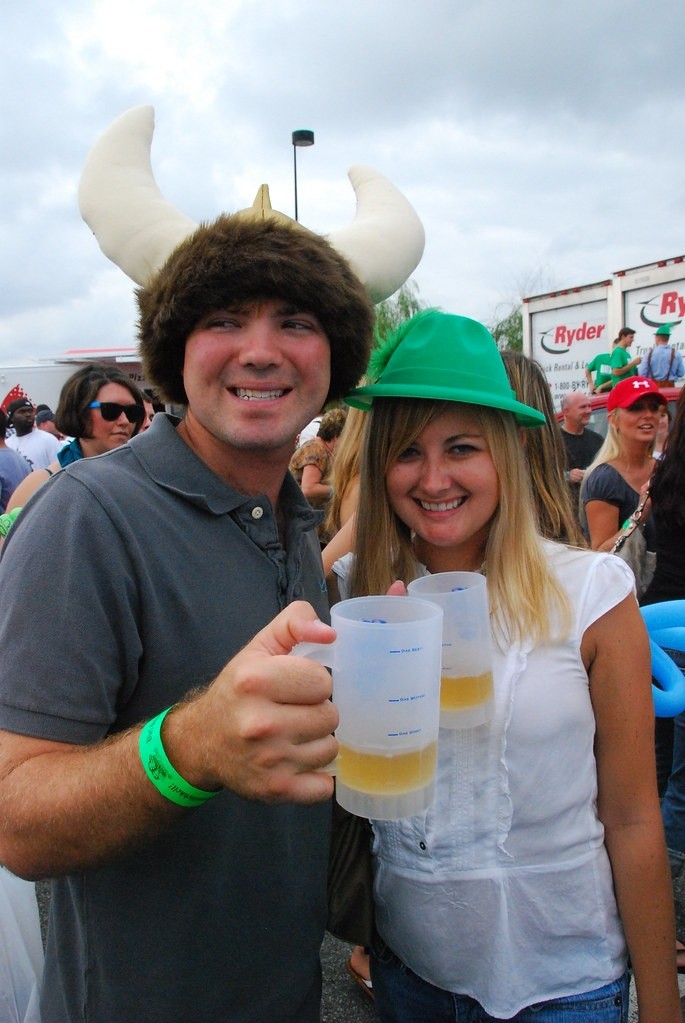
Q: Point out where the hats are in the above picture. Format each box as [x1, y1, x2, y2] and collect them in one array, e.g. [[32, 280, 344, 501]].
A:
[[341, 311, 550, 426], [606, 375, 671, 411], [78, 102, 425, 407], [6, 398, 33, 429], [654, 326, 672, 336], [36, 411, 57, 426]]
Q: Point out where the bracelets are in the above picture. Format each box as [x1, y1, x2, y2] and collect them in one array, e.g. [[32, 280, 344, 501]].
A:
[[622, 519, 630, 530], [564, 471, 570, 481], [329, 485, 334, 497], [136, 704, 219, 807]]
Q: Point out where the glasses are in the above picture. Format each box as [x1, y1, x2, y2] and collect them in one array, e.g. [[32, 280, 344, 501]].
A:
[[91, 401, 145, 424]]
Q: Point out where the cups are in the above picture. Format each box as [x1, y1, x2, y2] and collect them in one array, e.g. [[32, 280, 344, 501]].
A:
[[287, 595, 443, 820], [408, 569, 495, 730]]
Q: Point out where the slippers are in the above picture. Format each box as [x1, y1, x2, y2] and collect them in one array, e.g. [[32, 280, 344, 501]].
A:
[[346, 953, 378, 1005]]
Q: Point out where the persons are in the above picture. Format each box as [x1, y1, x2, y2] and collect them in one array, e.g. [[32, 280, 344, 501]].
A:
[[0, 214, 685, 1023]]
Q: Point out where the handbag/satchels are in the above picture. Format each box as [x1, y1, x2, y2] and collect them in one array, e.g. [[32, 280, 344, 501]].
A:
[[326, 800, 375, 948], [605, 480, 658, 599]]
